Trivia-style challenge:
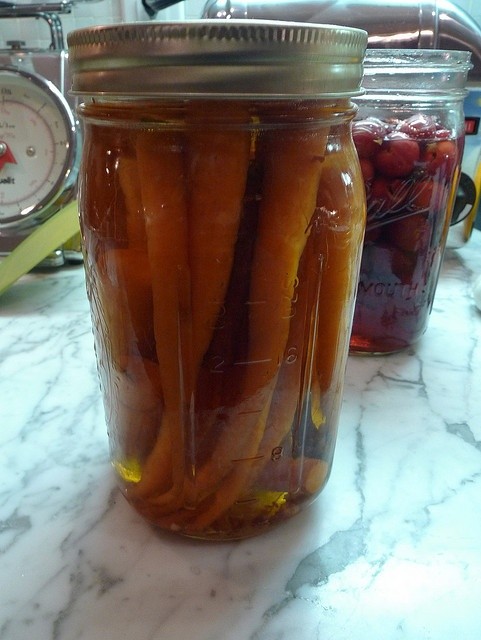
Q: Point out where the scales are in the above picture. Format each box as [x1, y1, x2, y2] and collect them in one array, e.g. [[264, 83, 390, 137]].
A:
[[0, 1, 105, 269]]
